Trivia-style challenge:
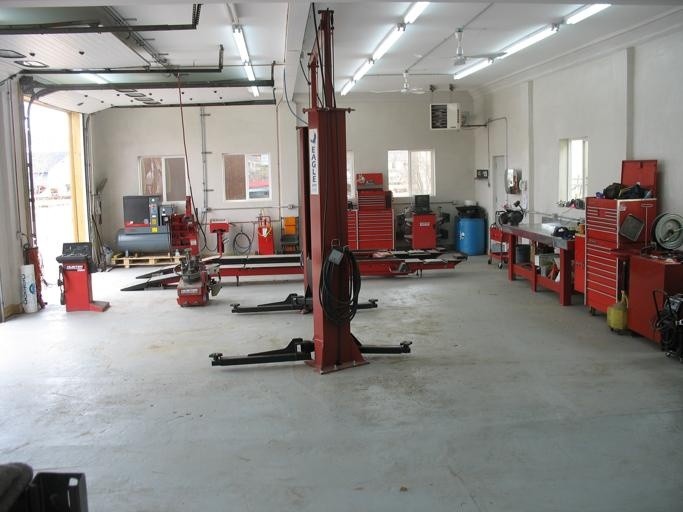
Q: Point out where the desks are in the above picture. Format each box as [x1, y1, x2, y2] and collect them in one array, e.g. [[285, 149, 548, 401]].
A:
[[500, 221, 573, 306]]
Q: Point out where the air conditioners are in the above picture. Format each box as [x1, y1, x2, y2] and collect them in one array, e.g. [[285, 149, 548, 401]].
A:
[[429, 101, 461, 132]]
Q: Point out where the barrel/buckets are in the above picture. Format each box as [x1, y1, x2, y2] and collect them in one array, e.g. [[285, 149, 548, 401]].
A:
[[514, 244, 531, 264], [455, 218, 485, 255]]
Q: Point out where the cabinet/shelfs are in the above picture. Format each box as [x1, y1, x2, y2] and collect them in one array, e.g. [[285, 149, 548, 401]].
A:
[[573, 159, 682, 344]]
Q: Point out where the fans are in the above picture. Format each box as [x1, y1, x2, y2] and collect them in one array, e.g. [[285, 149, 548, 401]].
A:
[[369, 27, 507, 96]]
